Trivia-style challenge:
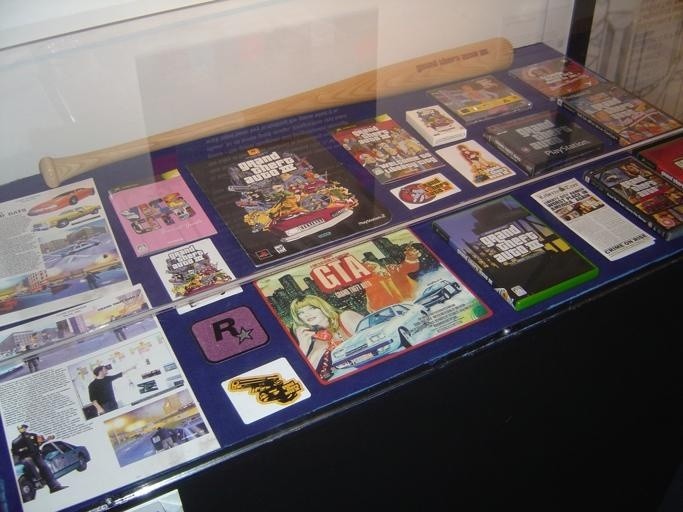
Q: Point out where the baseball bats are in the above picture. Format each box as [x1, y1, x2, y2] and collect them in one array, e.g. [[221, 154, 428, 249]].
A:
[[39, 37, 514, 189]]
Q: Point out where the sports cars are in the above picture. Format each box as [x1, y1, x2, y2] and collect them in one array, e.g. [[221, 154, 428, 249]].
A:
[[26, 187, 95, 217]]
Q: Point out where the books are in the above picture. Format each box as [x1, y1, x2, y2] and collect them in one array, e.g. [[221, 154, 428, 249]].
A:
[[0, 169, 312, 511], [185, 52, 683, 387]]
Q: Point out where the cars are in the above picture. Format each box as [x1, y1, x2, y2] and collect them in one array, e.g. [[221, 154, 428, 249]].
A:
[[412, 277, 460, 311], [269, 204, 353, 244], [331, 301, 431, 374], [11, 438, 91, 502], [30, 204, 100, 233]]
